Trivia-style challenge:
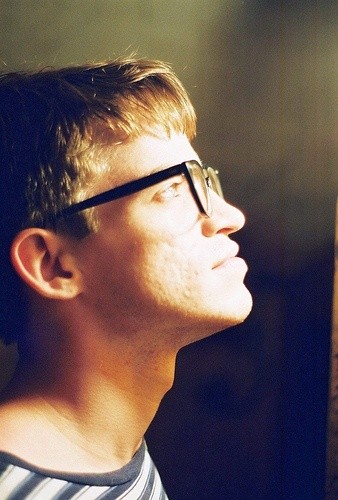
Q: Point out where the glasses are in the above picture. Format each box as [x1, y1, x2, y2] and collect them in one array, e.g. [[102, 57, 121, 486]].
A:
[[28, 160, 224, 217]]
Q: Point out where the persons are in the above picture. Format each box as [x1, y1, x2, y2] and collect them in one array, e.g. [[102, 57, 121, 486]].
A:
[[1, 48, 253, 500]]
